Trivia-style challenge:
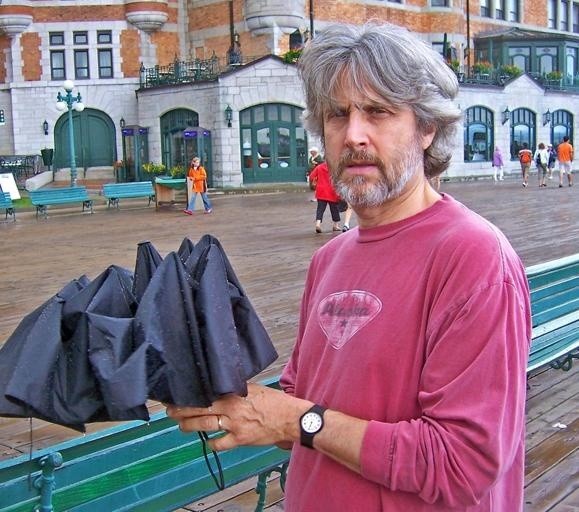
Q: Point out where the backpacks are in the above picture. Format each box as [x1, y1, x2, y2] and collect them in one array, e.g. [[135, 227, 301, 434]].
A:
[[519, 150, 529, 163]]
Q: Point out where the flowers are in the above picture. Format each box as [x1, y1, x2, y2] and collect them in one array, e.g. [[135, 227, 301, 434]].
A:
[[142, 161, 166, 173], [171, 163, 186, 177], [283, 45, 304, 66]]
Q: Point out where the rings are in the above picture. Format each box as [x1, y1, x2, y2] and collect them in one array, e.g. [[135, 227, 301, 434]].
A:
[[215, 414, 222, 431]]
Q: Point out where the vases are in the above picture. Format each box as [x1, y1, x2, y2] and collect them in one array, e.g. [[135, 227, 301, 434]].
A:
[[174, 173, 185, 179]]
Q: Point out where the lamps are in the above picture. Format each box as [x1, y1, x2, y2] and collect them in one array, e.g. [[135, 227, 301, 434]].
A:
[[44, 120, 50, 135], [225, 105, 233, 126], [234, 30, 242, 47], [120, 118, 126, 129], [303, 27, 310, 43], [501, 105, 511, 125], [542, 108, 551, 127]]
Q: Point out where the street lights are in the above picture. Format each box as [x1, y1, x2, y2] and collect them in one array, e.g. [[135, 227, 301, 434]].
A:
[[55, 79, 84, 187]]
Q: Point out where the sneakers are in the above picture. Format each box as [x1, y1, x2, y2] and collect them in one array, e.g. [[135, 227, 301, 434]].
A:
[[343, 225, 350, 232], [204, 208, 212, 214], [493, 178, 572, 187], [333, 227, 341, 231], [184, 210, 192, 215], [316, 226, 321, 233]]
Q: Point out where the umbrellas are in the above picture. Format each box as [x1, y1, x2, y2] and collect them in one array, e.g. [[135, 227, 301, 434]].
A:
[[1, 233, 282, 434]]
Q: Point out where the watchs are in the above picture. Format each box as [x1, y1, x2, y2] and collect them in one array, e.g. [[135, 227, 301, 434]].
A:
[[296, 402, 326, 451]]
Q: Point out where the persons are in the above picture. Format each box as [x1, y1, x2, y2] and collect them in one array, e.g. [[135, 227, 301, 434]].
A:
[[468, 132, 564, 161], [493, 137, 575, 191], [307, 145, 324, 191], [309, 157, 339, 234], [152, 18, 532, 511], [341, 201, 352, 233], [184, 156, 213, 216]]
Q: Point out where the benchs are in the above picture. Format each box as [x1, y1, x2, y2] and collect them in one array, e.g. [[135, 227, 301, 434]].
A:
[[521, 254, 579, 389], [29, 185, 94, 219], [103, 181, 157, 210], [0, 375, 303, 512], [0, 191, 17, 222]]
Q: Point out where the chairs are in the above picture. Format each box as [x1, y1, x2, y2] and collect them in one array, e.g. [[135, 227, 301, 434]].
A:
[[0, 155, 35, 177], [469, 72, 579, 94], [144, 61, 246, 88]]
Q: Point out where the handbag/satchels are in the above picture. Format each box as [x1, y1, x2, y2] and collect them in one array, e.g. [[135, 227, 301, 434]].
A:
[[337, 198, 348, 211], [536, 153, 540, 166]]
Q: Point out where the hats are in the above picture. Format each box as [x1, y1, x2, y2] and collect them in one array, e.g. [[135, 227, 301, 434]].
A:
[[547, 144, 552, 147], [308, 147, 318, 152]]
